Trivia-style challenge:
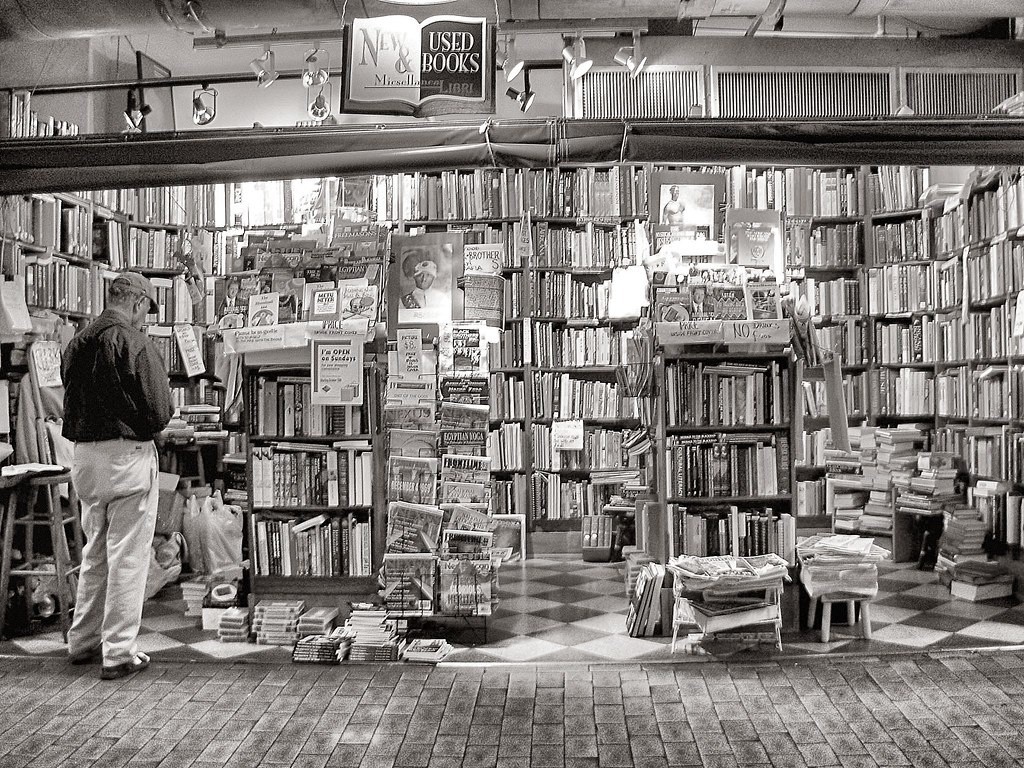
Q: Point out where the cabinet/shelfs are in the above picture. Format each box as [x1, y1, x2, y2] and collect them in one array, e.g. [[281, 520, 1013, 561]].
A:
[[0, 170, 1024, 637]]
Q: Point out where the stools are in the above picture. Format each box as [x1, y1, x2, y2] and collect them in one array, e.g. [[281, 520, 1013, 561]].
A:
[[809, 589, 872, 643], [165, 441, 225, 491], [0, 475, 81, 643]]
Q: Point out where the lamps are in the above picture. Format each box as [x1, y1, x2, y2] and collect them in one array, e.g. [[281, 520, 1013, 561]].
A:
[[124, 86, 152, 128], [301, 42, 330, 85], [505, 69, 535, 113], [614, 46, 646, 79], [562, 45, 593, 78], [307, 84, 333, 121], [192, 84, 216, 125], [496, 35, 524, 82], [248, 44, 279, 87]]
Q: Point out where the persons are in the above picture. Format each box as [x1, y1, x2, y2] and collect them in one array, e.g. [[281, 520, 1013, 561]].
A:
[[398, 261, 450, 310], [60, 273, 174, 679]]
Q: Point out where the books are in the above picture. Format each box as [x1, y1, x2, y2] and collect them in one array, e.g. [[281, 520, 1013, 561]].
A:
[[0, 90, 1024, 662], [340, 14, 497, 117]]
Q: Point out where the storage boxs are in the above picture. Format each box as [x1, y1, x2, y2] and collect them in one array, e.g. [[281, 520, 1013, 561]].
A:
[[581, 514, 612, 561], [950, 581, 1012, 601]]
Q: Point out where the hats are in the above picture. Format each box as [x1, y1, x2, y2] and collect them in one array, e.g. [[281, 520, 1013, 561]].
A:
[[111, 271, 161, 314], [413, 260, 437, 278]]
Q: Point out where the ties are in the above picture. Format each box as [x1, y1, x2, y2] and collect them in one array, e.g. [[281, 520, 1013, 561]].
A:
[[229, 300, 233, 307], [696, 305, 701, 316]]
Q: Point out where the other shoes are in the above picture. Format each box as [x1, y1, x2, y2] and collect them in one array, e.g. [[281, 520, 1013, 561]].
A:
[[100, 652, 151, 679], [68, 641, 101, 664]]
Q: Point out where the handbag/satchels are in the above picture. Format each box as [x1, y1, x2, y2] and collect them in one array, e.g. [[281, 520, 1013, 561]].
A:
[[143, 547, 181, 603], [183, 490, 244, 571]]
